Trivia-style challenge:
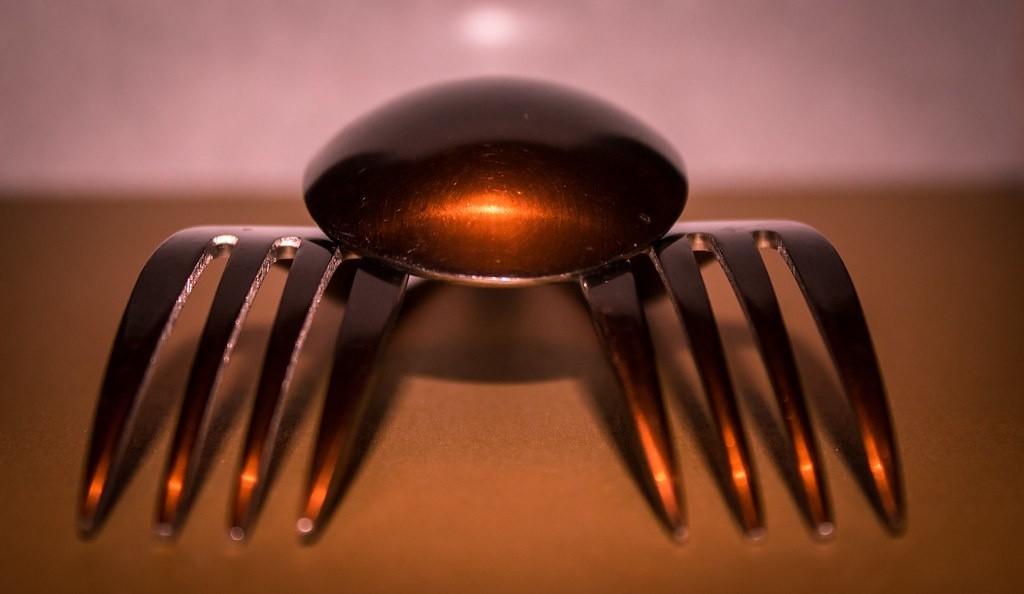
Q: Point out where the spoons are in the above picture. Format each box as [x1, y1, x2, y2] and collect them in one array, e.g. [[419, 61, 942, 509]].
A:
[[303, 77, 693, 288]]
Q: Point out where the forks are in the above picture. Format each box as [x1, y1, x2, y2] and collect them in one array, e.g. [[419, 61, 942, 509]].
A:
[[575, 222, 911, 540], [75, 225, 424, 536]]
